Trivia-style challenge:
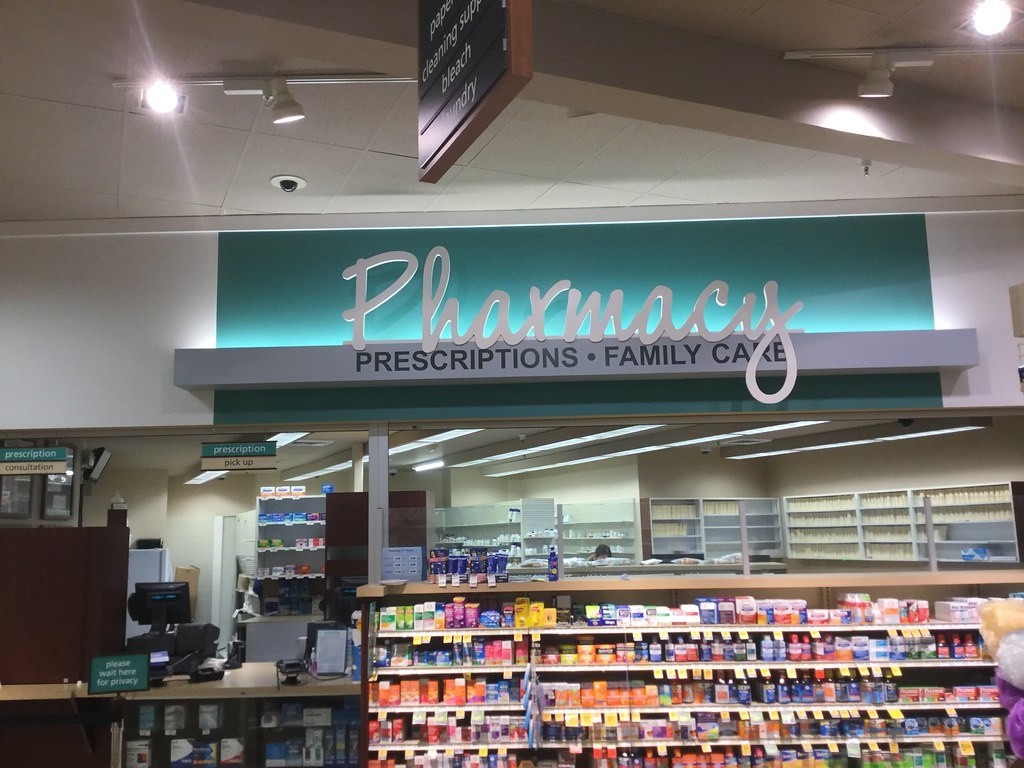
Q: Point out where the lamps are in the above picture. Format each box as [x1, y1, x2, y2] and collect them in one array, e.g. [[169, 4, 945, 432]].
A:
[[412, 458, 446, 471], [222, 75, 306, 125], [137, 79, 189, 120], [857, 51, 934, 98], [80, 443, 113, 484]]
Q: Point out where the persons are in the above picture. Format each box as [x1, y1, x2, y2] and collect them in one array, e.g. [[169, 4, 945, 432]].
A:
[[588, 544, 612, 561]]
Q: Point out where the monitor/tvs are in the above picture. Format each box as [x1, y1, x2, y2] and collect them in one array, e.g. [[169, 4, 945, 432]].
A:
[[134, 583, 192, 626]]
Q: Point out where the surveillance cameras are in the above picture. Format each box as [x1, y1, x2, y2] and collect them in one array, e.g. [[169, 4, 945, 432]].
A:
[[280, 180, 298, 192], [899, 418, 914, 428], [389, 469, 397, 476], [700, 445, 711, 455]]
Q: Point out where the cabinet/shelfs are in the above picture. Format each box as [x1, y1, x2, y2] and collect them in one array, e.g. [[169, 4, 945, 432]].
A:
[[359, 569, 1024, 768], [255, 494, 326, 580], [556, 497, 637, 567], [640, 497, 786, 565], [783, 481, 1024, 572], [433, 497, 560, 570]]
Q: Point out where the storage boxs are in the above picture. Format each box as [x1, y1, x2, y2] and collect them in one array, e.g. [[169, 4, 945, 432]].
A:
[[366, 677, 671, 709], [897, 684, 1003, 703], [369, 714, 679, 745], [834, 632, 906, 662], [683, 742, 1008, 768], [128, 707, 360, 768], [258, 483, 334, 577], [174, 565, 199, 623], [371, 639, 649, 668], [379, 596, 984, 632]]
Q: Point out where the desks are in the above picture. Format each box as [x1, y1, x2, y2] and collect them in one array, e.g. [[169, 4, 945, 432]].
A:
[[119, 658, 362, 702]]
